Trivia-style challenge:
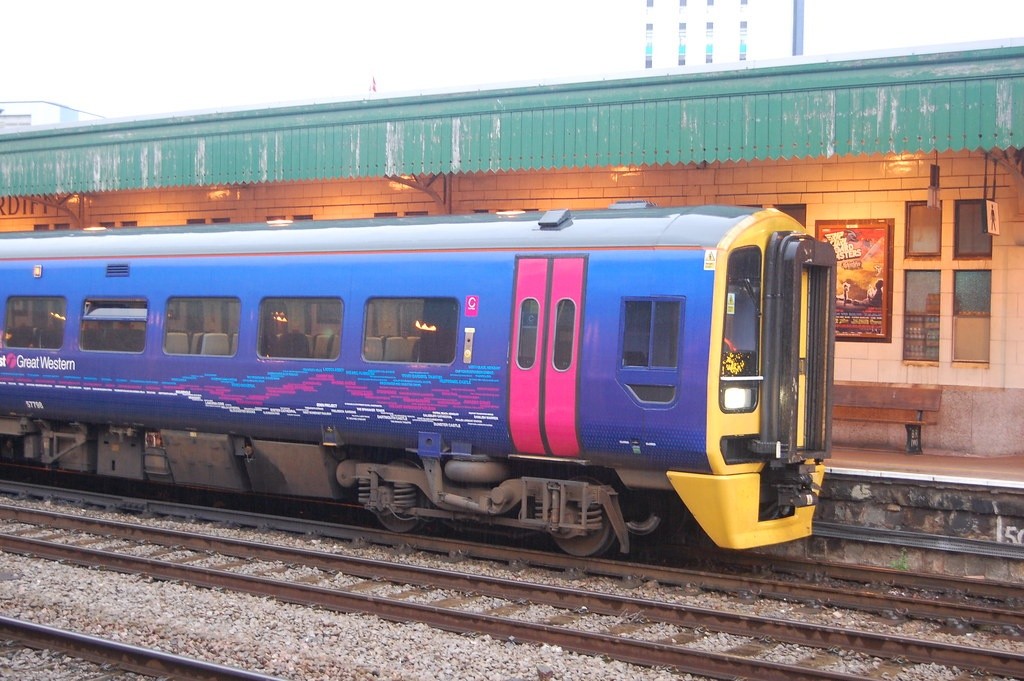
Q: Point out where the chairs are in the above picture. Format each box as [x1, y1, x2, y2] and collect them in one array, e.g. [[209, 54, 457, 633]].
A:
[[5, 323, 147, 353], [165, 330, 421, 363]]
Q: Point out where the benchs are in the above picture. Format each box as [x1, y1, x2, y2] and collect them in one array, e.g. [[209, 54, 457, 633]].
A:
[[832, 384, 941, 454]]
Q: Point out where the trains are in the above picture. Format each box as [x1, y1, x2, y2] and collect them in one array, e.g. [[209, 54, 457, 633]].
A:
[[0, 201, 839, 559]]
